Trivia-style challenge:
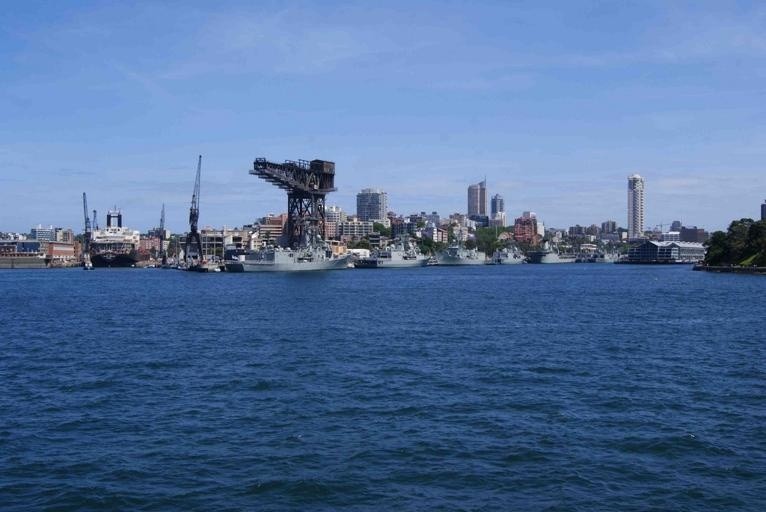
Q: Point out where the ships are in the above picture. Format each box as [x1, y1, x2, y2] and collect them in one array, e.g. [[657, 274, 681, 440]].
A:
[[90, 206, 150, 268]]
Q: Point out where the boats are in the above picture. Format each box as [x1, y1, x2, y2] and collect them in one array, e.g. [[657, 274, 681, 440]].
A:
[[500, 246, 529, 265], [240, 241, 356, 273], [591, 247, 624, 264], [433, 239, 486, 266], [354, 238, 433, 269], [540, 252, 577, 264]]
